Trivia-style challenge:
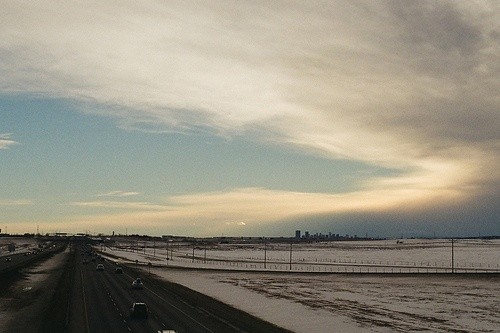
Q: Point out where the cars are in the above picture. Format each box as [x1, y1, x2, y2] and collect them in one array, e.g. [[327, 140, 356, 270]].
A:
[[95, 264, 105, 271], [132, 279, 144, 288]]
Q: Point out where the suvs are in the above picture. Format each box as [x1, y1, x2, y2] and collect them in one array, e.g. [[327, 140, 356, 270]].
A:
[[129, 303, 150, 319]]
